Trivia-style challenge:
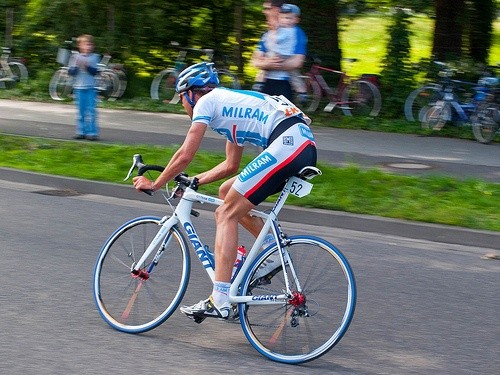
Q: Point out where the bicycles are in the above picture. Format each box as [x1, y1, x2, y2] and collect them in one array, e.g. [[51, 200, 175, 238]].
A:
[[291, 57, 384, 121], [48, 39, 131, 102], [92, 153, 358, 366], [402, 55, 500, 145], [150, 41, 241, 105], [0, 47, 29, 91]]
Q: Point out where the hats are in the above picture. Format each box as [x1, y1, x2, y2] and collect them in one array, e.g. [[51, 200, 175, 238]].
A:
[[280, 3, 301, 17]]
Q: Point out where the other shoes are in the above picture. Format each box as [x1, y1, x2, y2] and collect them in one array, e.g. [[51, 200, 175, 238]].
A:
[[295, 92, 307, 99], [73, 135, 85, 140], [252, 83, 266, 91], [86, 135, 97, 141]]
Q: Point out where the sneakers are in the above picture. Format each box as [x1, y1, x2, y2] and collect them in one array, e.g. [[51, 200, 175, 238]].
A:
[[251, 251, 291, 279], [179, 295, 232, 319]]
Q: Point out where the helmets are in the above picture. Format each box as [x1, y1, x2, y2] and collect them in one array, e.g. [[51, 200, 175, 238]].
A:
[[176, 62, 220, 93]]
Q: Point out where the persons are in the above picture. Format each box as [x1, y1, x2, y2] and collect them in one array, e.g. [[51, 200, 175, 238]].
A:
[[132, 62, 317, 318], [68, 34, 100, 140], [251, 0, 306, 104]]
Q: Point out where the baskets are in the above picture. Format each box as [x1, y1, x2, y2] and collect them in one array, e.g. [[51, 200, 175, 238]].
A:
[[55, 48, 71, 66]]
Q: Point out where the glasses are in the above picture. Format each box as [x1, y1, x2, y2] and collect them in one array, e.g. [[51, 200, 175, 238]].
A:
[[178, 87, 198, 100], [262, 6, 275, 11]]
